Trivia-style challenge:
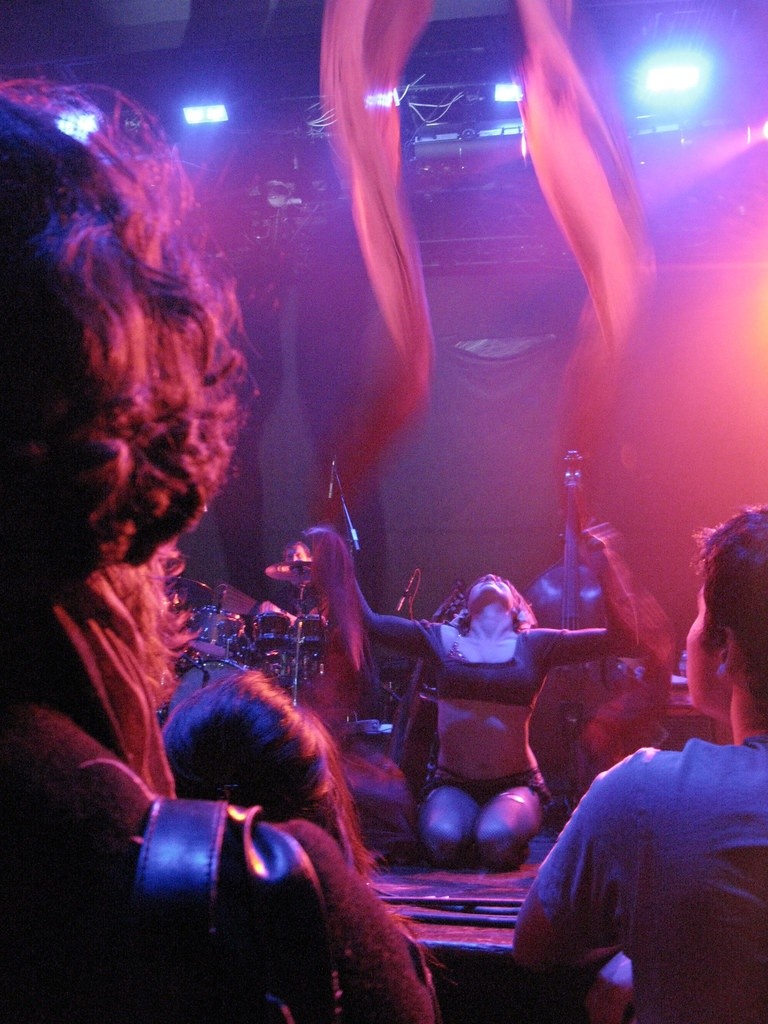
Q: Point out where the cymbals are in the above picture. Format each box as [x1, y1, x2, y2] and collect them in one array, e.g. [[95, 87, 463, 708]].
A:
[[262, 558, 319, 587]]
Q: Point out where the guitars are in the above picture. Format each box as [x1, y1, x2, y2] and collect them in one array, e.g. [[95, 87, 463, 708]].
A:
[[525, 450, 603, 629]]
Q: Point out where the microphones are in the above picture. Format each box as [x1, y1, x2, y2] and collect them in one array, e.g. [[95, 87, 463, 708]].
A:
[[329, 461, 335, 498], [395, 569, 418, 611]]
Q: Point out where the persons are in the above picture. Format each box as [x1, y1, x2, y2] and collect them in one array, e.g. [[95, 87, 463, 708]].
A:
[[293, 527, 372, 723], [0, 77, 437, 1023], [512, 506, 768, 1024], [155, 669, 367, 876], [298, 524, 640, 870]]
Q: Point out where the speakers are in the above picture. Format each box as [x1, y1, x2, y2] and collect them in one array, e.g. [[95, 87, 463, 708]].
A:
[[651, 702, 717, 753]]
